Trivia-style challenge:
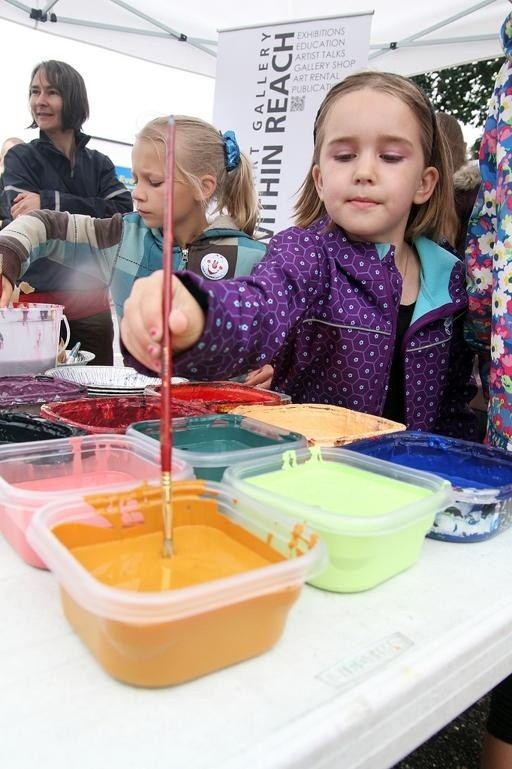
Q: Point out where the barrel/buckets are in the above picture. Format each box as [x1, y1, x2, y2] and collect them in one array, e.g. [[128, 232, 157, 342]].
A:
[[0, 302, 71, 379]]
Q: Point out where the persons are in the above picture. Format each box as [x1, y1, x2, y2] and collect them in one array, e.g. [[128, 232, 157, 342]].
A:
[[462, 8, 511, 460], [1, 137, 26, 172], [118, 70, 474, 439], [1, 115, 276, 392], [0, 59, 136, 234], [433, 111, 481, 254]]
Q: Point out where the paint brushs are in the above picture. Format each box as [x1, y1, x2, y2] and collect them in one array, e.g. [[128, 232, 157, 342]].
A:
[[159, 115, 176, 558]]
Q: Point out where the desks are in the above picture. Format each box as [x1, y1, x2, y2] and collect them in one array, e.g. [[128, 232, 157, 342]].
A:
[[0, 515, 512, 769]]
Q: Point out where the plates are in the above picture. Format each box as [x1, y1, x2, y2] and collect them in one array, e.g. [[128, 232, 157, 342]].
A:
[[44, 349, 191, 399]]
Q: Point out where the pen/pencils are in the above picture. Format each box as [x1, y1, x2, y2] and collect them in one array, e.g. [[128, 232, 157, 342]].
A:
[[0, 254, 4, 297]]
